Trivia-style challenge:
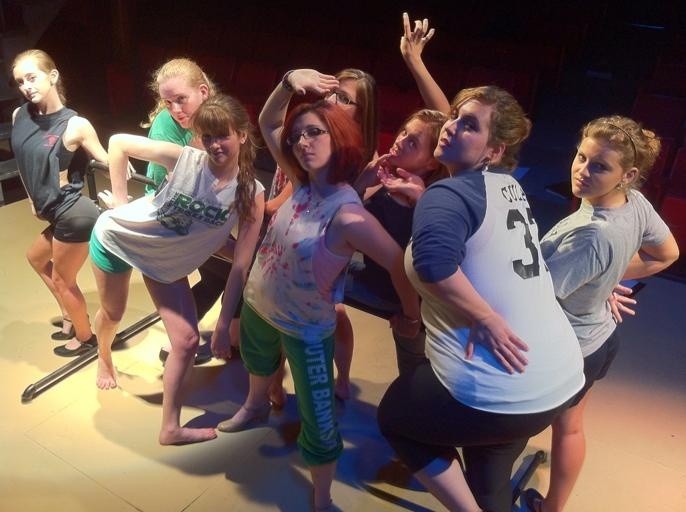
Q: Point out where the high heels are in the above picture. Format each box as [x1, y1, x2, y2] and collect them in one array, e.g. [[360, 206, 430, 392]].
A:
[[216, 399, 272, 435], [525, 484, 548, 512]]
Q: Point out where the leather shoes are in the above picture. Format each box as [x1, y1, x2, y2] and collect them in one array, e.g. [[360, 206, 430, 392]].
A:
[[52, 334, 98, 358], [50, 314, 89, 341]]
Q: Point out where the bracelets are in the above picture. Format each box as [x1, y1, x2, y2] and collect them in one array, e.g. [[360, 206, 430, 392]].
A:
[[281, 67, 295, 93], [402, 314, 423, 326]]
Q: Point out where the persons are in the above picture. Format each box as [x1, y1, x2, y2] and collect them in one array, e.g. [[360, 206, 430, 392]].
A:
[[531, 108, 681, 511], [215, 63, 425, 512], [86, 92, 267, 446], [7, 47, 137, 357], [370, 84, 587, 510], [350, 8, 454, 467], [258, 67, 381, 410], [138, 55, 251, 365]]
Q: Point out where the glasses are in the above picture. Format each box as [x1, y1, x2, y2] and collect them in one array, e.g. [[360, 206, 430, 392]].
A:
[[284, 127, 331, 146], [327, 89, 358, 107]]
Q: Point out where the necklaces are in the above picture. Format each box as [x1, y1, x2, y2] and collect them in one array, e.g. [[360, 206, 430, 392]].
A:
[[304, 183, 329, 216]]
